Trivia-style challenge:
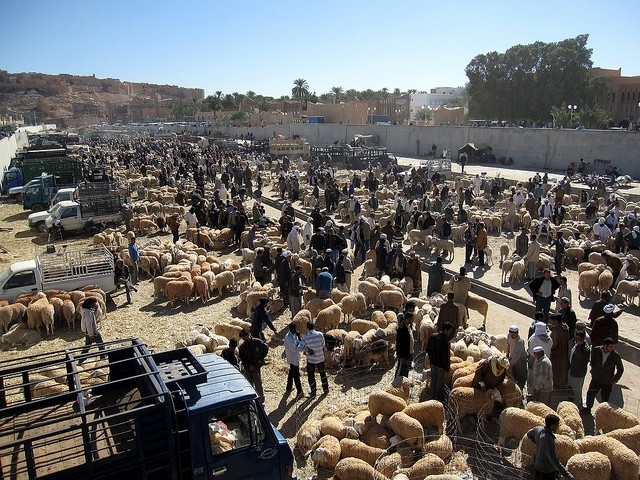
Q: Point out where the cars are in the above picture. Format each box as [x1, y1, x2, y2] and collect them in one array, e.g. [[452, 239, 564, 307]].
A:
[[91, 121, 212, 129], [10, 176, 47, 199], [26, 200, 70, 233]]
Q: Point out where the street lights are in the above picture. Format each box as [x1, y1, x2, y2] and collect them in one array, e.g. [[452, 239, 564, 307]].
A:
[[567, 104, 577, 122]]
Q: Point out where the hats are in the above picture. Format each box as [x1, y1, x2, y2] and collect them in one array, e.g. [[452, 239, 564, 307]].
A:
[[602, 304, 614, 313], [633, 225, 639, 231], [290, 226, 299, 234], [342, 250, 348, 253], [598, 217, 605, 224], [517, 190, 521, 195], [282, 251, 292, 257], [326, 249, 333, 252], [325, 220, 333, 226], [626, 254, 633, 260], [529, 192, 533, 196], [510, 325, 519, 332], [307, 217, 314, 222], [550, 314, 562, 323], [446, 293, 455, 298], [577, 321, 587, 327], [533, 346, 544, 352], [435, 212, 439, 217], [517, 180, 525, 184], [544, 198, 548, 202], [498, 356, 509, 370], [534, 322, 547, 335], [409, 251, 417, 257], [562, 297, 570, 303], [413, 205, 419, 208], [602, 338, 615, 344], [508, 185, 516, 190], [259, 298, 269, 303], [380, 234, 387, 239], [448, 202, 453, 206], [547, 192, 552, 195]]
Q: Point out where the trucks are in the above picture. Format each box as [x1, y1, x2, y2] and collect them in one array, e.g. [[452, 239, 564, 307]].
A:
[[418, 159, 454, 184], [43, 197, 123, 238], [49, 181, 119, 214], [347, 147, 398, 171], [268, 137, 311, 159], [314, 143, 363, 165], [350, 134, 379, 148], [1, 130, 82, 178], [1, 338, 299, 479], [21, 174, 111, 212], [2, 243, 118, 308]]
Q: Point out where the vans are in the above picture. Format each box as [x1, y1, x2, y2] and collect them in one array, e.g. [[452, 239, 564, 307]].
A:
[[304, 116, 326, 125]]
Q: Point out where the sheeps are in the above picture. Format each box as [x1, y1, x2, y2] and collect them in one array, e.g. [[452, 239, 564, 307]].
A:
[[297, 419, 321, 449], [575, 434, 639, 480], [449, 386, 503, 435], [338, 436, 388, 466], [310, 434, 342, 468], [335, 456, 390, 480], [93, 156, 639, 325], [565, 451, 612, 480], [366, 423, 390, 450], [594, 401, 640, 435], [520, 426, 580, 467], [367, 390, 407, 422], [0, 283, 106, 354], [173, 296, 513, 367], [421, 434, 453, 461], [385, 375, 413, 408], [386, 411, 425, 451], [400, 399, 444, 436], [527, 399, 576, 441], [556, 400, 586, 438], [497, 406, 548, 451], [321, 415, 359, 440], [606, 424, 640, 457], [406, 452, 446, 479], [23, 356, 115, 402]]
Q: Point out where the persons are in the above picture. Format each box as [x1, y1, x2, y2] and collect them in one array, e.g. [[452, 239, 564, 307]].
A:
[[474, 221, 487, 265], [525, 412, 576, 480], [527, 268, 560, 332], [283, 322, 305, 397], [526, 311, 551, 341], [582, 338, 625, 414], [303, 217, 353, 299], [525, 322, 553, 398], [278, 250, 299, 301], [622, 254, 637, 281], [83, 216, 101, 235], [449, 267, 472, 306], [349, 217, 423, 298], [25, 119, 376, 218], [471, 353, 510, 420], [274, 247, 283, 269], [376, 118, 640, 218], [168, 212, 181, 244], [248, 224, 259, 251], [261, 244, 272, 283], [590, 210, 640, 252], [566, 332, 590, 413], [530, 345, 555, 407], [250, 296, 279, 342], [587, 291, 626, 328], [590, 302, 619, 373], [548, 313, 570, 389], [221, 338, 241, 371], [252, 246, 267, 287], [425, 321, 454, 401], [553, 276, 572, 316], [503, 325, 528, 399], [77, 298, 107, 364], [439, 218, 452, 256], [238, 327, 269, 407], [297, 321, 330, 397], [523, 234, 540, 282], [127, 236, 141, 286], [571, 318, 593, 362], [287, 265, 311, 320], [558, 296, 577, 360], [287, 225, 301, 254], [601, 251, 623, 290], [534, 217, 555, 243], [473, 219, 480, 256], [278, 210, 292, 235], [516, 226, 528, 257], [395, 301, 415, 332], [281, 218, 293, 241], [464, 221, 475, 263], [394, 313, 415, 380], [436, 292, 459, 335], [548, 231, 567, 275], [113, 258, 138, 305], [426, 256, 447, 296]]
